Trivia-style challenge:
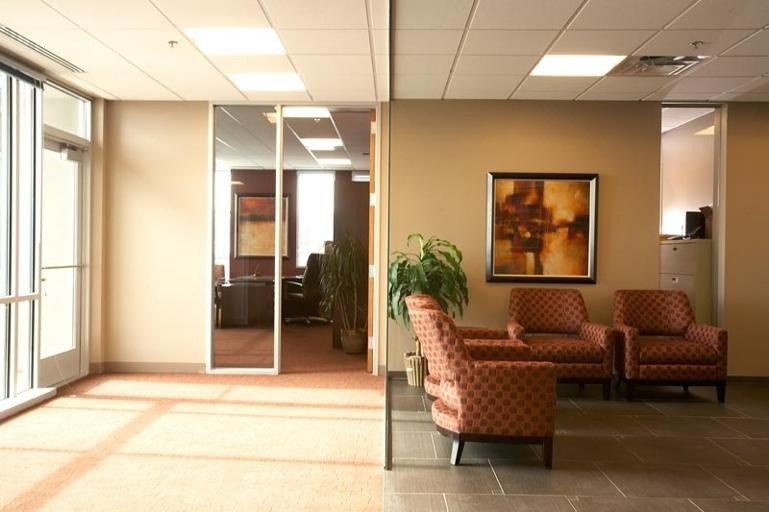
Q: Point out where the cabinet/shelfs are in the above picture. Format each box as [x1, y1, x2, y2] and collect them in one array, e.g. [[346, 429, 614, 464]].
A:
[[660, 238, 715, 323], [220, 283, 267, 329]]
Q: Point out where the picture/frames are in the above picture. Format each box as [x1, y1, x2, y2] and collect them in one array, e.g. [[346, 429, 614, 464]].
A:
[[481, 168, 602, 286], [233, 191, 291, 261]]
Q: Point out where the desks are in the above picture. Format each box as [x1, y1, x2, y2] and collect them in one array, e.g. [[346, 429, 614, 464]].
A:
[[228, 273, 303, 283]]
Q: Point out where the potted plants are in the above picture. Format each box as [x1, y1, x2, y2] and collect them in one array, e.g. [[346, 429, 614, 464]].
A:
[[386, 228, 469, 388], [312, 230, 370, 356]]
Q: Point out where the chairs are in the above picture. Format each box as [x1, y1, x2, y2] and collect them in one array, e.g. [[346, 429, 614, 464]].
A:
[[283, 253, 333, 327]]
